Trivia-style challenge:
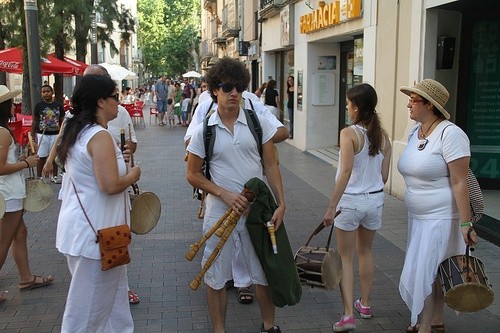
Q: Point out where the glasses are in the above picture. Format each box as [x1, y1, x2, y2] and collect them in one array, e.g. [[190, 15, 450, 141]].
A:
[[212, 82, 248, 92], [409, 98, 425, 104], [107, 93, 119, 102]]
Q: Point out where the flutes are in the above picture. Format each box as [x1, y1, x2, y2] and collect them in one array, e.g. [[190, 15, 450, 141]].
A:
[[182, 177, 302, 309]]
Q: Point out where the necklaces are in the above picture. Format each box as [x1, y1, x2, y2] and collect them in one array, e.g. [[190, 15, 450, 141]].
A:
[[420, 117, 439, 138]]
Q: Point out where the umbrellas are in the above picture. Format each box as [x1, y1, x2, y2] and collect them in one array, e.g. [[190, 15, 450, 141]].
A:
[[182, 71, 201, 77], [0, 46, 139, 85]]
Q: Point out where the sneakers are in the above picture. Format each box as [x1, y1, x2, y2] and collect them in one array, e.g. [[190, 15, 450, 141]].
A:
[[354, 297, 372, 318], [333, 313, 356, 331]]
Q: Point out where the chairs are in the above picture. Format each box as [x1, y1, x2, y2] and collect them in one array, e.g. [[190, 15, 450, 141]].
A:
[[120, 101, 158, 128], [9, 100, 72, 156]]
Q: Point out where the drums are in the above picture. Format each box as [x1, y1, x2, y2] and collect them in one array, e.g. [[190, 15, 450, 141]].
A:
[[22, 177, 53, 213], [0, 193, 7, 221], [128, 188, 162, 236], [438, 255, 496, 313], [293, 244, 344, 292]]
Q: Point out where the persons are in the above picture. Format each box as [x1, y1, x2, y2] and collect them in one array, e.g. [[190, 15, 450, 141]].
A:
[[286, 76, 294, 139], [397, 79, 478, 333], [0, 57, 288, 333], [323, 84, 393, 332]]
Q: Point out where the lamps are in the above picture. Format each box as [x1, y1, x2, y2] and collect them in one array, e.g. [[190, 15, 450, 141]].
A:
[[305, 0, 313, 10]]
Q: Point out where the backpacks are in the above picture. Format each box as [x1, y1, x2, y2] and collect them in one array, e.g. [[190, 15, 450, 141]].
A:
[[184, 85, 196, 98], [411, 123, 484, 223]]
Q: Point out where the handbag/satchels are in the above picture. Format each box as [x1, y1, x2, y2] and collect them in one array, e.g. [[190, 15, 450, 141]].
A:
[[260, 88, 267, 106], [98, 224, 131, 271]]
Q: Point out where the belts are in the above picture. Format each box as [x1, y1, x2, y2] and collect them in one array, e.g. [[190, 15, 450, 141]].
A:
[[369, 189, 384, 194]]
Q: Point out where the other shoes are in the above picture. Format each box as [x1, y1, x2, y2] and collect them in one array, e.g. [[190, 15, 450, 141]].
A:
[[289, 136, 293, 139], [50, 177, 62, 184], [260, 322, 281, 333], [38, 176, 46, 183], [127, 292, 139, 303]]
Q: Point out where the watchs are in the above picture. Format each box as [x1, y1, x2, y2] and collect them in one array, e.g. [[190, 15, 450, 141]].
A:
[[460, 221, 473, 227]]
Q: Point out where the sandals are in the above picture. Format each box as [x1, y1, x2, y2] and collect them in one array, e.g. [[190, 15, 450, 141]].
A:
[[1, 295, 6, 302], [19, 275, 55, 290], [226, 279, 235, 291], [407, 323, 445, 333], [239, 286, 253, 305]]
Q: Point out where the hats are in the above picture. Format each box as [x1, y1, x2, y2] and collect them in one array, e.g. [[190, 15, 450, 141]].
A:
[[400, 79, 450, 119], [0, 85, 22, 103]]
